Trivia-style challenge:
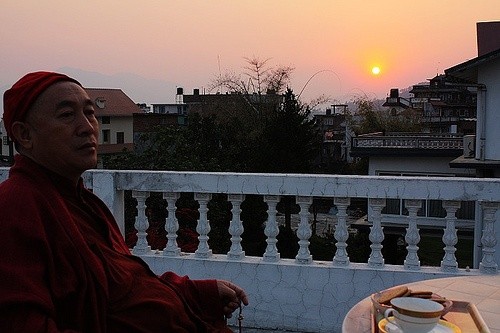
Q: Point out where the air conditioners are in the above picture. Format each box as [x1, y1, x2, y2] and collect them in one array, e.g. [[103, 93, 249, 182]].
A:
[[463, 134, 475, 158]]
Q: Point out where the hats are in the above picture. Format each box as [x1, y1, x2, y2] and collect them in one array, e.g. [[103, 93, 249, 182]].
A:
[[3, 72, 90, 138]]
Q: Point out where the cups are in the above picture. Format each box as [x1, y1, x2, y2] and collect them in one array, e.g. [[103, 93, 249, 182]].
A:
[[384, 298, 444, 333]]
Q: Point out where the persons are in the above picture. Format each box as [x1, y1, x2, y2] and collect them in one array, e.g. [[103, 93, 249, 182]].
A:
[[1, 72, 249, 332]]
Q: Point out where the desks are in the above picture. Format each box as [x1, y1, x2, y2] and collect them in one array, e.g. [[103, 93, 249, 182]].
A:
[[341, 275, 500, 333]]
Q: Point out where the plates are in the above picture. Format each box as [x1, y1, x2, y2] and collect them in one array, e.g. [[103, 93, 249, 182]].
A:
[[378, 317, 462, 333]]
